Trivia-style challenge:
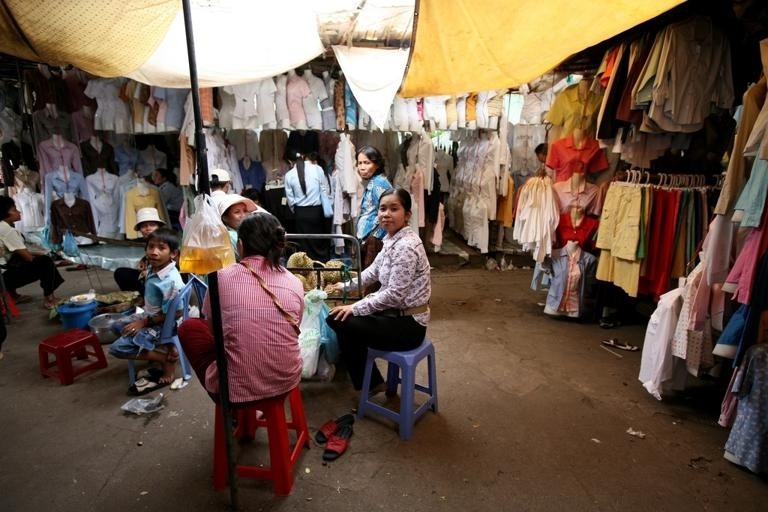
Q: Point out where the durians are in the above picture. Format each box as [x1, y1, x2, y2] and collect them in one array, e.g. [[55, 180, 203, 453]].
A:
[[287, 252, 364, 309]]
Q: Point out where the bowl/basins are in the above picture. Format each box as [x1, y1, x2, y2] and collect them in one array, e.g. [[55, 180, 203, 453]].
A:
[[69, 293, 95, 306]]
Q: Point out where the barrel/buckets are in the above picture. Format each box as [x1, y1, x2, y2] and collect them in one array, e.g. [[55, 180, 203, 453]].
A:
[[57, 301, 98, 330]]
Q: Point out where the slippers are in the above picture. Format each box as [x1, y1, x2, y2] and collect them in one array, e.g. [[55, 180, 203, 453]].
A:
[[322, 424, 352, 460], [315, 414, 354, 444], [128, 368, 170, 396]]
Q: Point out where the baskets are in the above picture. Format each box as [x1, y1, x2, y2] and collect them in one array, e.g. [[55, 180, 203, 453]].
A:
[[96, 301, 133, 314]]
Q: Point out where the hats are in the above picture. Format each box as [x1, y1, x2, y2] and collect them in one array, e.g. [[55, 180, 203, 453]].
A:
[[133, 207, 166, 231], [208, 169, 230, 181], [217, 196, 257, 217]]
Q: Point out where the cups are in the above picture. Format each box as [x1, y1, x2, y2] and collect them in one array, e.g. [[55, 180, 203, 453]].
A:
[[87, 290, 96, 299]]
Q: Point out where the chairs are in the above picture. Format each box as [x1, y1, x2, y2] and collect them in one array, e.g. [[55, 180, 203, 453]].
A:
[[188, 272, 210, 318], [125, 278, 194, 385]]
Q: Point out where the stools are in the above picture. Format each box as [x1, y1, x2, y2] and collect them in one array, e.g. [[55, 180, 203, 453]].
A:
[[1, 287, 20, 322], [33, 327, 110, 384], [210, 385, 313, 501], [357, 334, 442, 442]]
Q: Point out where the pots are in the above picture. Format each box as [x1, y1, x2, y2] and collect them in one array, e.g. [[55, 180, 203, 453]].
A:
[[87, 312, 125, 343]]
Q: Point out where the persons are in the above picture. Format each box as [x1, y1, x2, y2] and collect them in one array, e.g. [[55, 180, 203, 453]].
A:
[[180, 134, 432, 230], [208, 167, 258, 264], [280, 145, 335, 266], [544, 77, 610, 319], [323, 189, 432, 394], [0, 195, 66, 310], [534, 143, 546, 163], [0, 134, 202, 246], [108, 228, 187, 383], [36, 67, 549, 133], [114, 207, 171, 295], [353, 146, 396, 297], [176, 212, 306, 409]]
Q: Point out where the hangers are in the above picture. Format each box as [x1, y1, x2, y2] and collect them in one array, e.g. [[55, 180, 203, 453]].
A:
[[615, 167, 724, 193]]
